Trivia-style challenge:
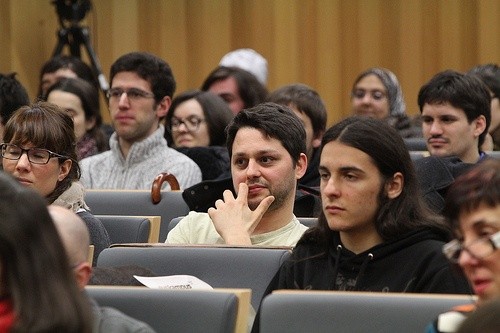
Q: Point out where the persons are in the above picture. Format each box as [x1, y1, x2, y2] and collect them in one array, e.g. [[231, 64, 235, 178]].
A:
[[45, 78, 106, 162], [250, 116, 475, 333], [164, 103, 310, 248], [422, 159, 500, 333], [350, 66, 423, 138], [0, 101, 111, 268], [465, 62, 500, 151], [41, 56, 99, 101], [45, 204, 91, 288], [267, 83, 327, 189], [0, 71, 30, 168], [0, 169, 156, 333], [78, 52, 203, 191], [411, 69, 500, 218], [200, 48, 269, 118], [164, 91, 231, 148]]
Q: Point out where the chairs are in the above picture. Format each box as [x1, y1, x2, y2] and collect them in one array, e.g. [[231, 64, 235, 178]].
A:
[[84, 151, 500, 333]]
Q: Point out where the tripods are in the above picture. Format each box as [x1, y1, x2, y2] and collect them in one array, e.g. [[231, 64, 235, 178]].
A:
[[51, 26, 109, 104]]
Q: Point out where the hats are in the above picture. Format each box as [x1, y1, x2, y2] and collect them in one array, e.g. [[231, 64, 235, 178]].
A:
[[220, 48, 268, 89]]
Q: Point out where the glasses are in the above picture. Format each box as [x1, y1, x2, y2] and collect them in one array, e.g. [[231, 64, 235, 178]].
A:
[[166, 116, 207, 132], [0, 143, 64, 165], [106, 88, 155, 101], [442, 231, 500, 263]]
